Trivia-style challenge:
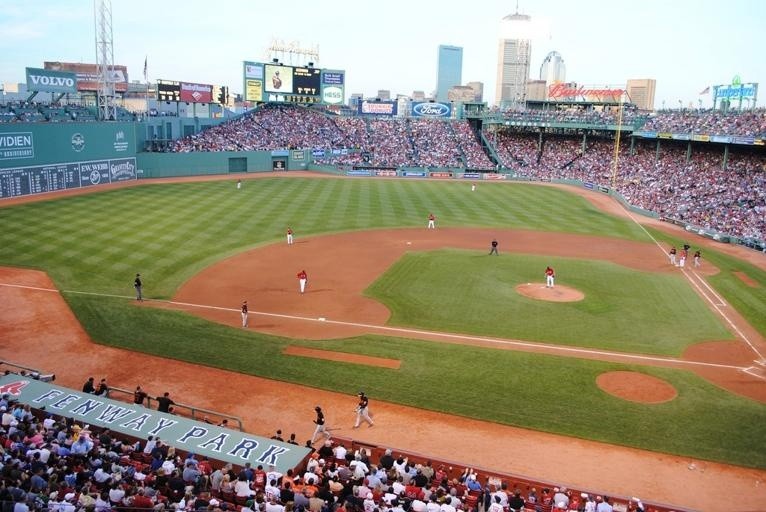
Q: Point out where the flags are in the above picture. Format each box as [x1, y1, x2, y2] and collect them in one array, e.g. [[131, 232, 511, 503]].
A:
[[141, 58, 148, 79]]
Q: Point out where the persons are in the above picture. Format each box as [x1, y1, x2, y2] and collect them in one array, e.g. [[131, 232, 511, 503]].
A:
[[694, 250, 702, 267], [134, 273, 143, 300], [272, 71, 282, 93], [95, 378, 108, 398], [1, 97, 178, 123], [298, 269, 308, 293], [83, 376, 95, 392], [544, 265, 556, 287], [287, 226, 294, 245], [142, 102, 765, 180], [488, 238, 499, 255], [427, 214, 436, 228], [472, 181, 476, 191], [679, 248, 686, 266], [588, 180, 766, 243], [134, 385, 149, 405], [236, 177, 241, 189], [0, 392, 667, 512], [670, 246, 677, 265], [240, 299, 248, 327]]
